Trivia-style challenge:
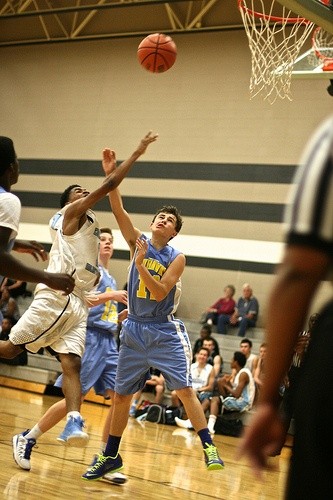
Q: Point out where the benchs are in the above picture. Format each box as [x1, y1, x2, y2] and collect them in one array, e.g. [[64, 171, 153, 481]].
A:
[[0, 349, 62, 395], [163, 318, 264, 427]]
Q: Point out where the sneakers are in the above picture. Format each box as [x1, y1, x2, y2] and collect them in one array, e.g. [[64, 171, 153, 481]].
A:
[[12, 429, 36, 471], [175, 416, 193, 428], [56, 415, 89, 448], [82, 452, 124, 480], [129, 401, 138, 415], [207, 425, 216, 433], [203, 442, 224, 470], [90, 453, 127, 484]]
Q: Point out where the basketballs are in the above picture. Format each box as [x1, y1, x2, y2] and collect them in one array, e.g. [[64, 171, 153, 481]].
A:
[[136, 32, 177, 74]]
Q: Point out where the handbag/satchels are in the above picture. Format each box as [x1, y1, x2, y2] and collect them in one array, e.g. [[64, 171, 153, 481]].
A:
[[146, 405, 181, 426], [213, 416, 244, 437]]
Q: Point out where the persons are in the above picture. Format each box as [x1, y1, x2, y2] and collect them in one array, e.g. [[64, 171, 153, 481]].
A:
[[0, 136, 75, 366], [0, 132, 158, 447], [82, 149, 225, 480], [13, 229, 129, 485], [129, 283, 318, 434], [235, 73, 333, 500], [0, 276, 27, 366]]
